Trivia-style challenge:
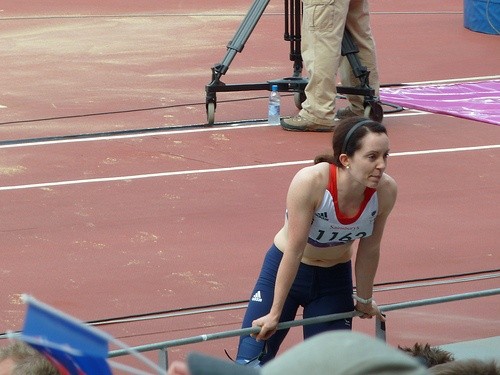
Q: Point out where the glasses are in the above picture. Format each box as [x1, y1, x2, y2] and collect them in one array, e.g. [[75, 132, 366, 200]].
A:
[[224, 338, 268, 364]]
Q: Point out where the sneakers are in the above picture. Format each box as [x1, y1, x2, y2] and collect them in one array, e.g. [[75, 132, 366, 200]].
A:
[[280, 113, 333, 133], [335, 106, 366, 120]]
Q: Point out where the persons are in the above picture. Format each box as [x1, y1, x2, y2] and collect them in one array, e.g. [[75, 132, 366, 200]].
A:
[[280, 0, 380, 131], [0, 329, 500, 375], [235, 116, 397, 367]]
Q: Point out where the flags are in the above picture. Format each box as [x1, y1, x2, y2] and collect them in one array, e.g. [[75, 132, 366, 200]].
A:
[[10, 303, 111, 375]]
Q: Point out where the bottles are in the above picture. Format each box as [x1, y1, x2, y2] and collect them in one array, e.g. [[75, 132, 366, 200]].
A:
[[268, 84, 280, 125]]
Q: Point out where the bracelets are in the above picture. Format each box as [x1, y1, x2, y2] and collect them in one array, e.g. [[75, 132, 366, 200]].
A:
[[351, 294, 373, 304]]
